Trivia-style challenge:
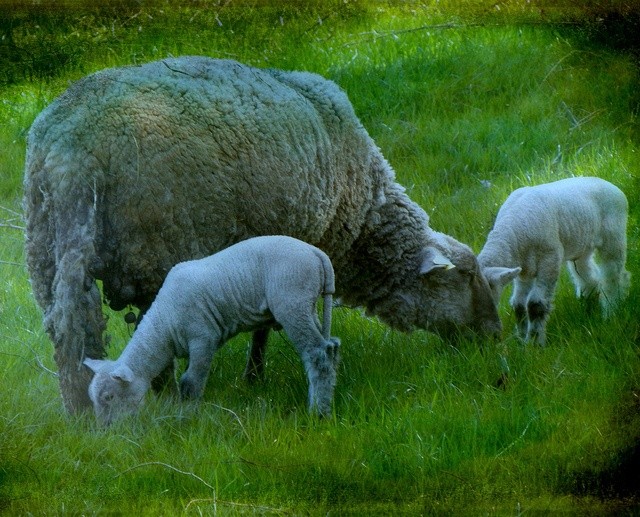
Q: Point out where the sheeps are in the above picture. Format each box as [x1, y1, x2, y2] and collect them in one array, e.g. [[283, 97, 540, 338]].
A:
[[20, 54, 511, 428], [478, 175, 629, 346], [84, 235, 337, 437]]
